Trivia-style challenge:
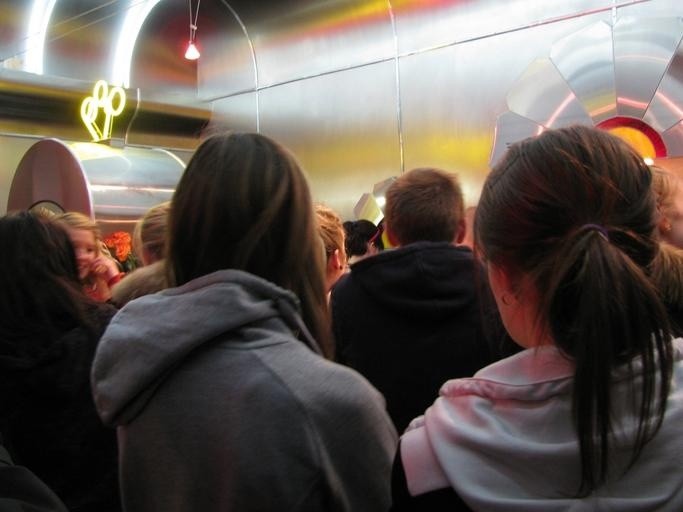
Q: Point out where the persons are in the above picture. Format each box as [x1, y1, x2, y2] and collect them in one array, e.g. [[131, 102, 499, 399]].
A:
[[1, 129, 683, 512]]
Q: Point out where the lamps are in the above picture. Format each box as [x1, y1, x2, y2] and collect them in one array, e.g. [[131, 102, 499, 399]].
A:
[[183, 0, 202, 61]]
